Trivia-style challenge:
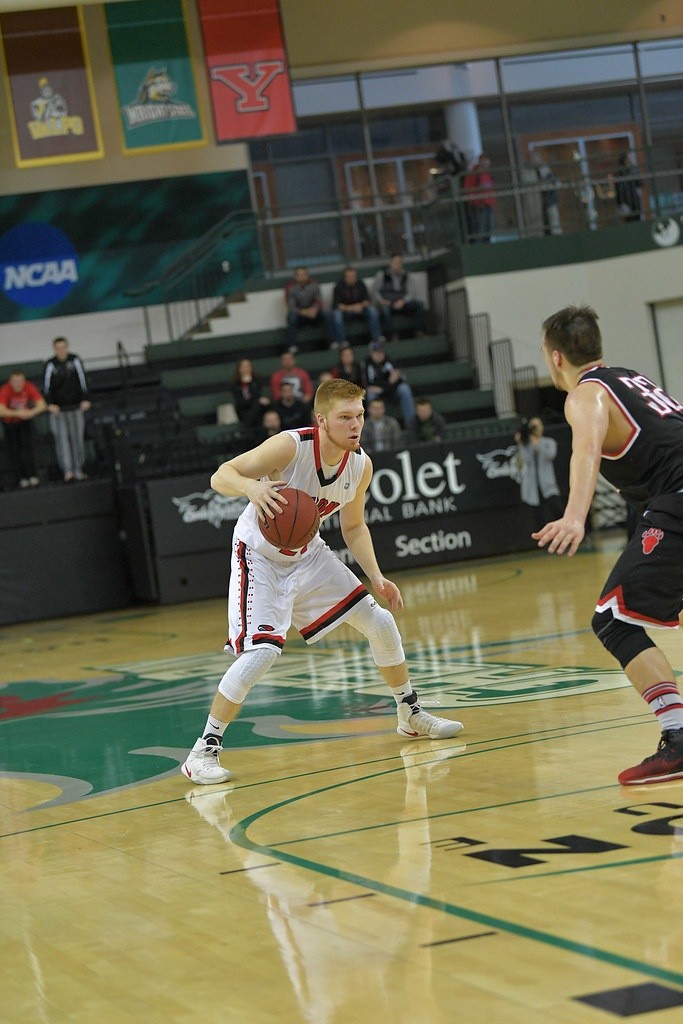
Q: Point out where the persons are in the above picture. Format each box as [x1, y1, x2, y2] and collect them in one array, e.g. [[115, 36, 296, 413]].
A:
[[231, 340, 451, 453], [0, 370, 47, 488], [514, 418, 566, 532], [435, 132, 498, 246], [43, 336, 92, 481], [533, 154, 557, 237], [530, 307, 683, 785], [332, 268, 387, 350], [179, 379, 464, 785], [371, 254, 426, 340], [284, 265, 339, 354], [614, 152, 644, 222]]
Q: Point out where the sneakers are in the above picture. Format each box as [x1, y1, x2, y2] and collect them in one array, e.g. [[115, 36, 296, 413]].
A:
[[618, 726, 683, 785], [399, 738, 467, 780], [396, 702, 464, 739], [185, 782, 235, 824], [181, 737, 230, 785]]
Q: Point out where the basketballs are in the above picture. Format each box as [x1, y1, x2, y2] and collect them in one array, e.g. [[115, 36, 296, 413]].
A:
[[259, 488, 320, 550]]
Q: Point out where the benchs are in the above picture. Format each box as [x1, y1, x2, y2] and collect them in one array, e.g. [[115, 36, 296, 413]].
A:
[[0, 360, 98, 475], [142, 309, 528, 467]]
